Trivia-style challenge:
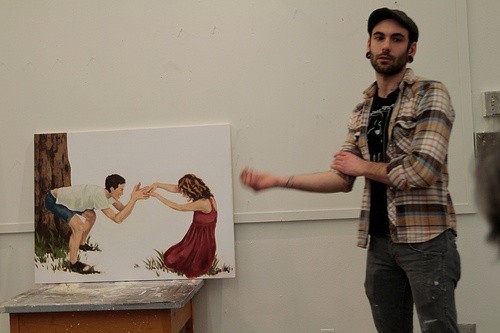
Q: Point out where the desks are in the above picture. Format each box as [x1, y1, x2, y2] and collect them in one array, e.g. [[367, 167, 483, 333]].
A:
[[0, 282, 208, 333]]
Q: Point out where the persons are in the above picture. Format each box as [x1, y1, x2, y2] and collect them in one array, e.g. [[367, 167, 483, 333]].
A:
[[237, 8, 464, 333]]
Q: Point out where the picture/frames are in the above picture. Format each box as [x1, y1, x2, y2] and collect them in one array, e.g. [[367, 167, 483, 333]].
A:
[[34, 121, 240, 281]]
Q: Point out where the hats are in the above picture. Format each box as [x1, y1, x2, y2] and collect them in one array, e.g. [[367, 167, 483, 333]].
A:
[[368, 7, 419, 42]]
